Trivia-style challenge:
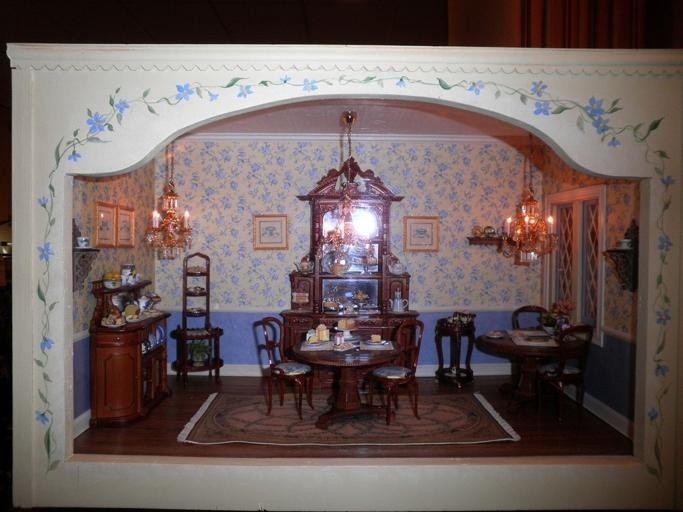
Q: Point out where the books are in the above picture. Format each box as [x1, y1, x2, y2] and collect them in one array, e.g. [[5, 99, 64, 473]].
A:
[[507, 328, 559, 348]]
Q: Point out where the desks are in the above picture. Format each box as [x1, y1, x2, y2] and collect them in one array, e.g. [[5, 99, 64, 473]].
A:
[[290, 335, 401, 430], [476, 327, 583, 406], [433, 317, 474, 389]]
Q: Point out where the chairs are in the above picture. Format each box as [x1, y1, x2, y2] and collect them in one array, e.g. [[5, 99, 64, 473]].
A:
[[261, 316, 315, 420], [535, 324, 596, 427], [367, 319, 425, 427], [513, 304, 551, 331]]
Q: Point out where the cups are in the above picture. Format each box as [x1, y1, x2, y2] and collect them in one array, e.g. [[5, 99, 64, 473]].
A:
[[77, 235, 90, 247]]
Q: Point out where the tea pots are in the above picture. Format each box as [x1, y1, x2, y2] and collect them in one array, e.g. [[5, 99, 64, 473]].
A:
[[133, 295, 154, 313], [141, 323, 166, 355], [388, 260, 407, 276], [389, 287, 409, 313], [293, 254, 314, 275]]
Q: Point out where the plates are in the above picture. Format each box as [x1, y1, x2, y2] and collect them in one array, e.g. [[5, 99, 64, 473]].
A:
[[485, 333, 505, 340], [184, 269, 205, 314], [74, 246, 92, 249], [102, 310, 160, 329], [304, 327, 389, 352]]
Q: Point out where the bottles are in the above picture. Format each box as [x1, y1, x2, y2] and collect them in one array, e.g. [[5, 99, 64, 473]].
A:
[[101, 263, 141, 290]]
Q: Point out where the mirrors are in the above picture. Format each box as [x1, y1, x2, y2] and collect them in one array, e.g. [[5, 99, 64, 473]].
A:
[[287, 158, 411, 312]]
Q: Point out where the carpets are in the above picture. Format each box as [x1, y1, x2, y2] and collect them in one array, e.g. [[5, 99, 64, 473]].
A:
[[175, 389, 523, 448]]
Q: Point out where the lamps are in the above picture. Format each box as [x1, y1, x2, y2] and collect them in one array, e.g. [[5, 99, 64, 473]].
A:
[[494, 132, 561, 258], [142, 140, 193, 259], [316, 109, 374, 258]]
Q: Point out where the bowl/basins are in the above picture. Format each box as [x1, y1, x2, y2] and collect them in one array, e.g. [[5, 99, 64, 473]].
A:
[[540, 324, 556, 335]]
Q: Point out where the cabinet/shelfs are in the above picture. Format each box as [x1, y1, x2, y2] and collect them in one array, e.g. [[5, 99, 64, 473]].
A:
[[283, 314, 417, 388], [181, 251, 211, 328], [168, 326, 224, 388], [88, 312, 171, 423]]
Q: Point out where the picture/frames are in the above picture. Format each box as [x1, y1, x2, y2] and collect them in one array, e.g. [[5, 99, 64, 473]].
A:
[[93, 201, 117, 247], [117, 205, 136, 248], [252, 214, 289, 250], [402, 216, 439, 250]]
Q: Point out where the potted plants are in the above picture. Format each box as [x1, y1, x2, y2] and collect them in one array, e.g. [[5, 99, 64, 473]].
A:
[[186, 339, 212, 362]]
